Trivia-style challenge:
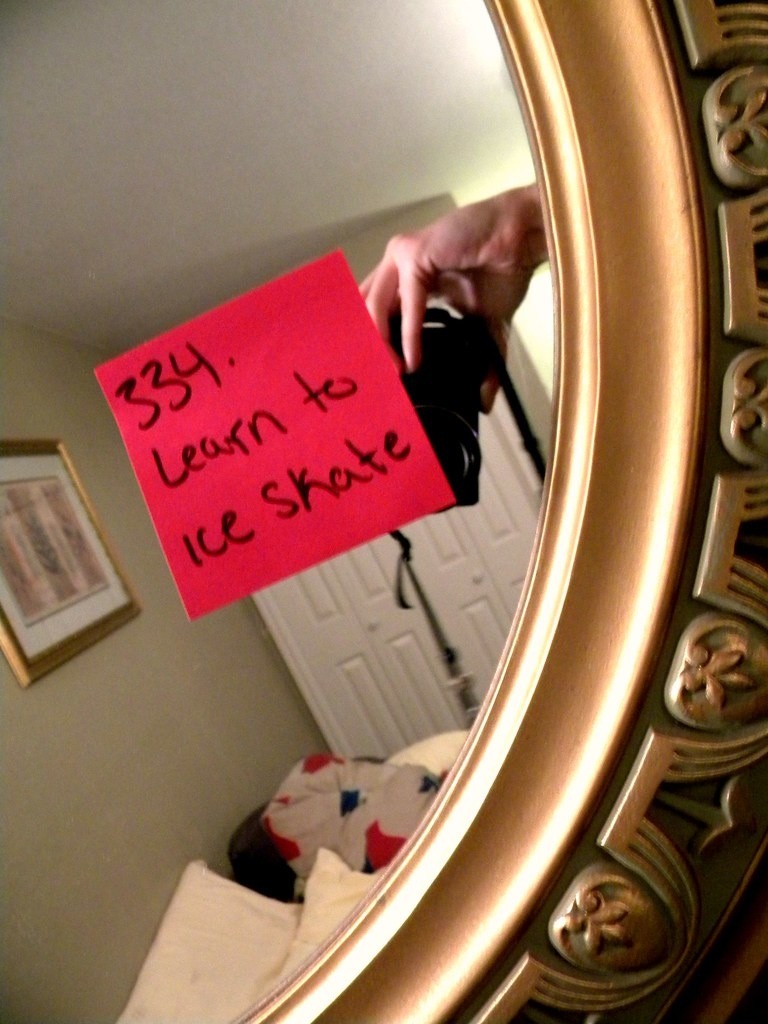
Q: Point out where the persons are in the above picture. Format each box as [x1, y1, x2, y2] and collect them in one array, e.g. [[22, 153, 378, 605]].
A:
[[360, 181, 551, 415]]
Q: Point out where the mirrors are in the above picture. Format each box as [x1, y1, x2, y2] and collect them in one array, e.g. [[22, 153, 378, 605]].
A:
[[0, 0, 768, 1024]]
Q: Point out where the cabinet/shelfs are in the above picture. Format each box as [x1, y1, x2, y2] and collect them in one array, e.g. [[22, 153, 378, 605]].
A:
[[250, 389, 541, 760]]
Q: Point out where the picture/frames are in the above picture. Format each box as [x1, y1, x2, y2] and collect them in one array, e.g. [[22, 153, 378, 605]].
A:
[[0, 437, 142, 692]]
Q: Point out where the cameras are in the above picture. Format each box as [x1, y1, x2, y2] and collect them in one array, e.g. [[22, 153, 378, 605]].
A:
[[384, 307, 483, 507]]
